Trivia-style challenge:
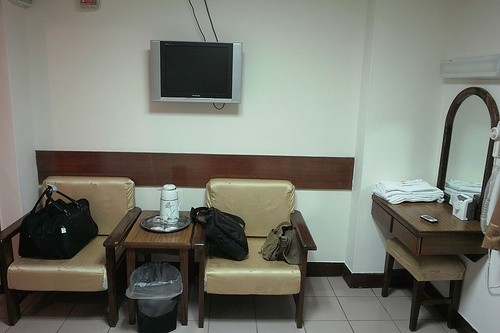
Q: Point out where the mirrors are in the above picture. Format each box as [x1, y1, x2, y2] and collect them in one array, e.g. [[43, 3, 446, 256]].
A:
[[439, 86, 500, 222]]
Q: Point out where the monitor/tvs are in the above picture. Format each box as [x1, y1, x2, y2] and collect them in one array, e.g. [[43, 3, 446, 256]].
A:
[[148, 39, 243, 105]]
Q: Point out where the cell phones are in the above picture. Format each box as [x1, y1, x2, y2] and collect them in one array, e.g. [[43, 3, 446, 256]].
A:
[[420, 214, 438, 223]]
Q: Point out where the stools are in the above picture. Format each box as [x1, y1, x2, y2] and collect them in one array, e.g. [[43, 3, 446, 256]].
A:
[[382, 237, 466, 331]]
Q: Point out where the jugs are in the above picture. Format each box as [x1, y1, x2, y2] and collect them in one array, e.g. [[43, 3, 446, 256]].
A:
[[157, 183, 183, 224]]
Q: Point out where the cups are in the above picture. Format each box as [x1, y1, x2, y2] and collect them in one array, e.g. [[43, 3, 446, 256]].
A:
[[151, 216, 167, 231], [167, 216, 183, 231]]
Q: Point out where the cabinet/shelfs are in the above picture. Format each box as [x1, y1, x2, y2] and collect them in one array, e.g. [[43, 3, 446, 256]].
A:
[[371, 194, 489, 256]]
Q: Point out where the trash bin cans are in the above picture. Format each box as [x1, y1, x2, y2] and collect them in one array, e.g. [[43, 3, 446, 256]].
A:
[[131, 262, 182, 332]]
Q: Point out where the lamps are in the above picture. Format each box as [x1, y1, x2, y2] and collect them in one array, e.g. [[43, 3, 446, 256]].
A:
[[438, 55, 500, 80]]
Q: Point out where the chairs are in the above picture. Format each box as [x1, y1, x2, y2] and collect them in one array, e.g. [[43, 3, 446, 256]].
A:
[[0, 175, 142, 327], [192, 177, 317, 328]]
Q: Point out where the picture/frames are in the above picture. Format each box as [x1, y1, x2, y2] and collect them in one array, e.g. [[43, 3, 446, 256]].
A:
[[77, 0, 102, 12]]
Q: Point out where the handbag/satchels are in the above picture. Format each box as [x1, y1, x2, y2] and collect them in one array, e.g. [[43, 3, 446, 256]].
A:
[[18, 184, 98, 260], [258, 222, 300, 265], [190, 206, 249, 262]]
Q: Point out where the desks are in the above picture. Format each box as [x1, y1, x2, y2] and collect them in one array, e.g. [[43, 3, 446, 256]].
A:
[[122, 210, 195, 325]]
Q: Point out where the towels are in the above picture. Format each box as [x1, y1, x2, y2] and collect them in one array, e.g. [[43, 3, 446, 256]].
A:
[[373, 178, 445, 205], [445, 179, 482, 195]]
